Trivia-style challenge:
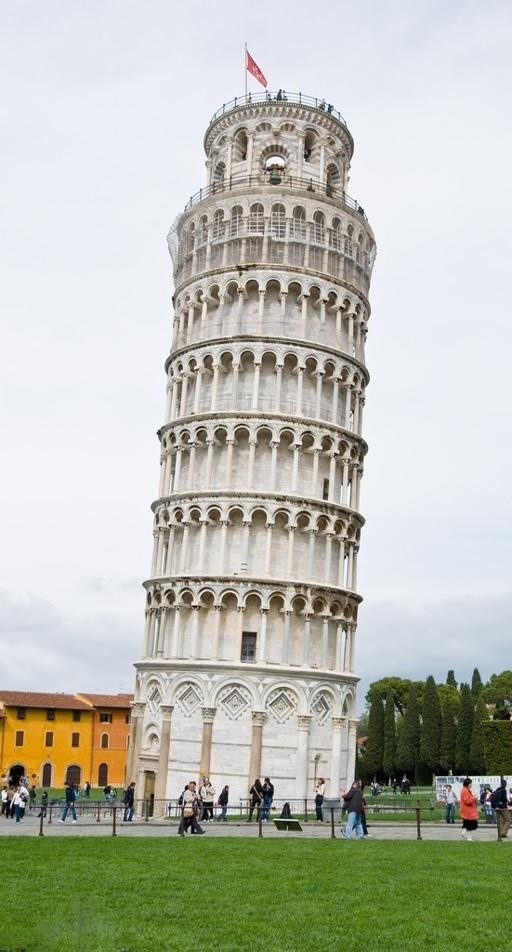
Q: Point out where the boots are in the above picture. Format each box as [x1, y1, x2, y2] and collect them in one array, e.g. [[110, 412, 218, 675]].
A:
[[459, 828, 509, 842]]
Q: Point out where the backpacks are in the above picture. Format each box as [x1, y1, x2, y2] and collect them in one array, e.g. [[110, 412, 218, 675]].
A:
[[120, 790, 128, 802], [488, 787, 504, 808]]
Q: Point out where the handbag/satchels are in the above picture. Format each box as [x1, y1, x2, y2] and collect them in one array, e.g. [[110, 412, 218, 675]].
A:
[[183, 805, 195, 819]]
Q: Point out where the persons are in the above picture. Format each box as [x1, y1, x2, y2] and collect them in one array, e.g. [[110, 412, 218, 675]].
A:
[[443, 777, 511, 842], [371, 777, 411, 796], [103, 781, 136, 821], [277, 89, 283, 100], [1, 773, 91, 824], [178, 776, 229, 835], [249, 778, 276, 823], [318, 102, 326, 110], [327, 104, 333, 112], [312, 779, 372, 839]]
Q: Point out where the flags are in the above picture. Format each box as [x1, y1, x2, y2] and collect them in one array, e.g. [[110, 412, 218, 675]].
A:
[[246, 51, 268, 87]]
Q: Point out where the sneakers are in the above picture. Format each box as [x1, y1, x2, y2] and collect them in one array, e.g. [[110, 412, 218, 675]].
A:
[[340, 829, 373, 839], [71, 819, 79, 823], [177, 817, 267, 837], [58, 819, 65, 824]]
